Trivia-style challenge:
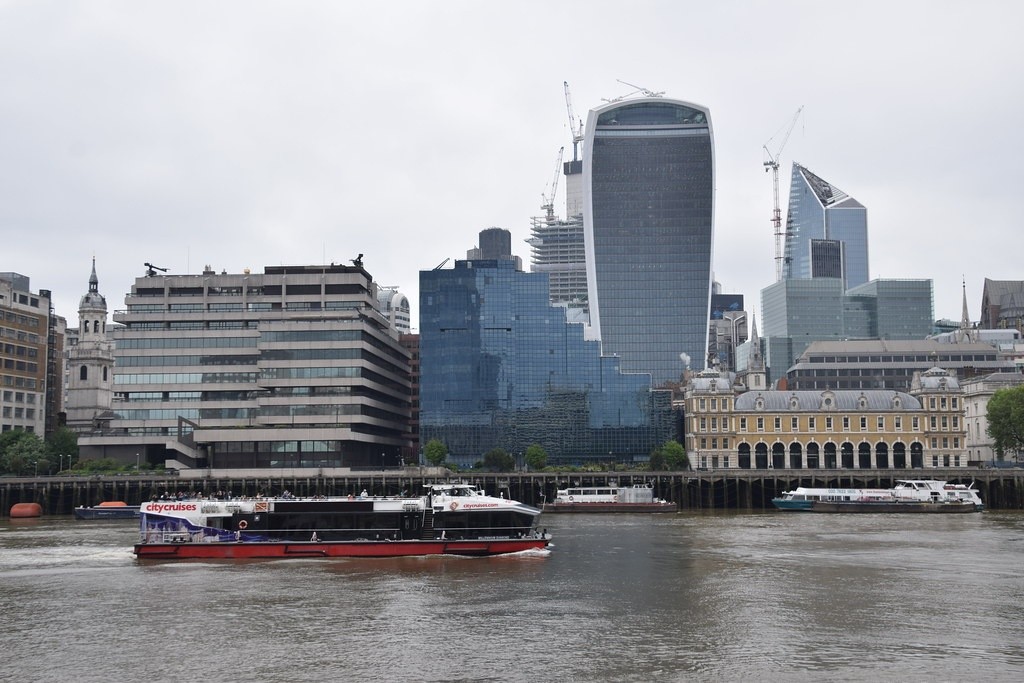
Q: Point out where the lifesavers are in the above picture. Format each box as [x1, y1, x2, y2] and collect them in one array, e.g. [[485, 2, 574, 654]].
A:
[[614, 496, 617, 500], [239, 520, 248, 529], [569, 496, 573, 501]]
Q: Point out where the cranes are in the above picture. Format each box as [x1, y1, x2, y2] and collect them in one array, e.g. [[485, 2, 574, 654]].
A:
[[540, 146, 569, 223], [760, 103, 808, 281], [601, 77, 665, 105], [560, 77, 587, 143]]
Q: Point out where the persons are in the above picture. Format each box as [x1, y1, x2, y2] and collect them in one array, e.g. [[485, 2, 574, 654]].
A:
[[403, 490, 409, 498], [348, 491, 353, 500], [361, 489, 368, 497], [152, 490, 325, 500]]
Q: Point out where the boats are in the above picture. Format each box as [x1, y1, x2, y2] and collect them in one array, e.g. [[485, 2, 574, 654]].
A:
[[770, 479, 985, 513], [537, 485, 679, 513], [131, 482, 554, 564], [73, 501, 142, 520]]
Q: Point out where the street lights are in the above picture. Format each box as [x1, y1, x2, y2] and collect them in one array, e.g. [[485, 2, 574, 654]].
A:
[[519, 452, 523, 471], [59, 454, 62, 471], [381, 453, 384, 472], [136, 454, 139, 472], [34, 462, 37, 477], [609, 451, 612, 472], [68, 455, 71, 477]]
